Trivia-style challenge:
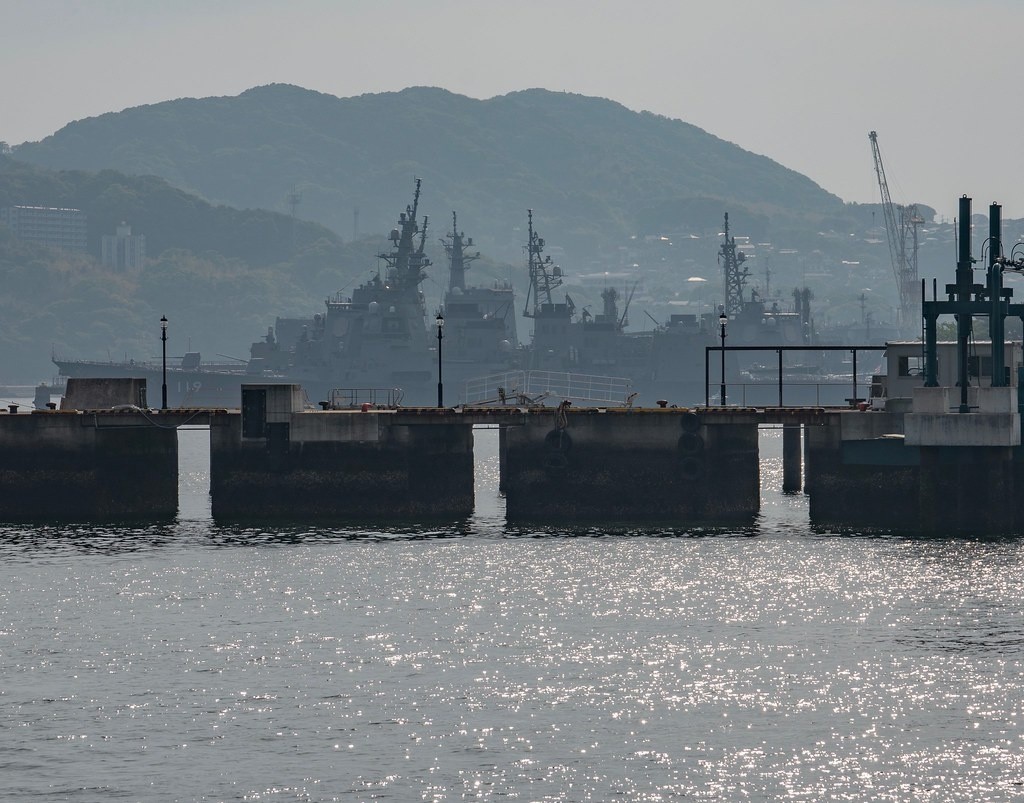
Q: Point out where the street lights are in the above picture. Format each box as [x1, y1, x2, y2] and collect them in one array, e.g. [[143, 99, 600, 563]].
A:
[[720, 311, 728, 407], [435, 314, 446, 407], [160, 313, 170, 408]]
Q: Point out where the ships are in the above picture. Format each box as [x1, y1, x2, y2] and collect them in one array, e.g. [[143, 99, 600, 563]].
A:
[[523, 195, 890, 416], [53, 176, 521, 408]]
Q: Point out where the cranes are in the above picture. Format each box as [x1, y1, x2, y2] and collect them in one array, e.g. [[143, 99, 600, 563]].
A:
[[867, 128, 926, 343]]
